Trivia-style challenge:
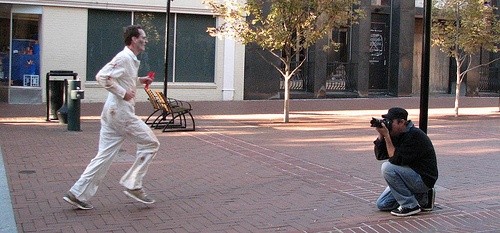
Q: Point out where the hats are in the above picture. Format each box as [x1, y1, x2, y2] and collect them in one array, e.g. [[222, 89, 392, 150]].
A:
[[381, 107, 408, 120]]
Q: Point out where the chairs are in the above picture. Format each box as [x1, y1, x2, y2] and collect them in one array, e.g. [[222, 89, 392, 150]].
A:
[[144, 87, 195, 132]]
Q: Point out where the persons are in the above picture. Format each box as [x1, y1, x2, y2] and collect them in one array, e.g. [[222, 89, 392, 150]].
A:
[[374, 108, 439, 217], [62, 25, 161, 210]]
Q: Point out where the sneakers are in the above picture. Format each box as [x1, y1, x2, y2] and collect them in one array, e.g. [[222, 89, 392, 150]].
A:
[[391, 204, 421, 216], [63, 191, 94, 209], [422, 188, 436, 211], [123, 188, 155, 204]]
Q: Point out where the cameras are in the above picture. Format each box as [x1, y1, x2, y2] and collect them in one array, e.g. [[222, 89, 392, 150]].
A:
[[370, 117, 391, 133]]
